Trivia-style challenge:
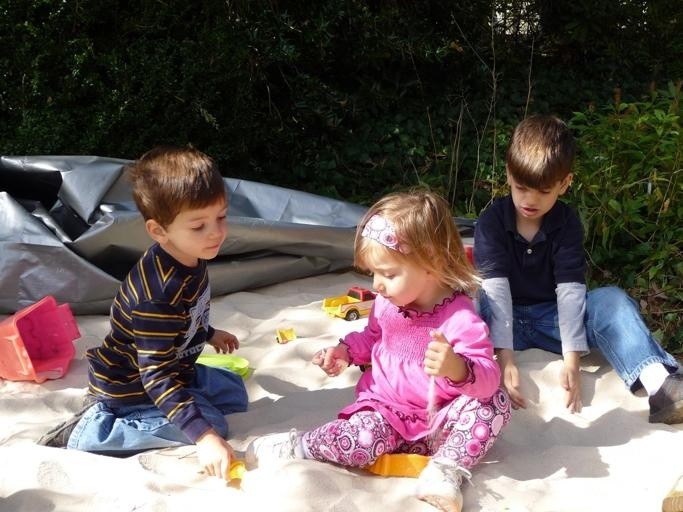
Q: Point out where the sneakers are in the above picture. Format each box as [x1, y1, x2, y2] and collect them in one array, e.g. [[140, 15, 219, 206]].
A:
[[415, 457, 463, 512], [649, 373, 683, 425], [245, 428, 306, 470], [37, 402, 96, 447]]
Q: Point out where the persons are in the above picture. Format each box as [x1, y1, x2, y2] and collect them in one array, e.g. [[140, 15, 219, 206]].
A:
[[473, 111, 683, 425], [36, 141, 249, 482], [244, 181, 512, 512]]
